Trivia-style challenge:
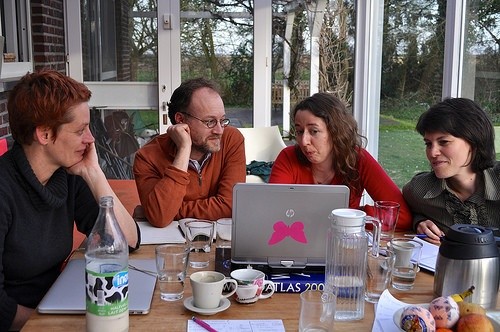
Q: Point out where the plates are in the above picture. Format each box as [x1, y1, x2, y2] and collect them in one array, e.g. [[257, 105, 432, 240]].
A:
[[183, 296, 232, 315]]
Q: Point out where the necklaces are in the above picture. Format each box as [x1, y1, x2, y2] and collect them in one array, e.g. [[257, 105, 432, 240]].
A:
[[311, 166, 336, 186]]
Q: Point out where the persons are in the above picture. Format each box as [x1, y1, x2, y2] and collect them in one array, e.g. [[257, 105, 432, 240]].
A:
[[403, 96, 500, 243], [268, 93, 415, 233], [0, 69, 143, 332], [132, 78, 246, 228]]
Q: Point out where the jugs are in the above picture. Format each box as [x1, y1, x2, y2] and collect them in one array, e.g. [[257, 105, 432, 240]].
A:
[[324, 208, 382, 322], [434, 223, 500, 311]]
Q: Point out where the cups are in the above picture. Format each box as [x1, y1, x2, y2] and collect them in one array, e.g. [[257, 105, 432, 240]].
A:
[[217, 218, 233, 248], [230, 269, 277, 304], [154, 244, 190, 301], [185, 221, 215, 268], [365, 201, 423, 303], [190, 270, 238, 309], [299, 289, 336, 332]]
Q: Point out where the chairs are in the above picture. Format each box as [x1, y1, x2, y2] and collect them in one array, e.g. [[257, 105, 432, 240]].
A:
[[234, 125, 288, 184]]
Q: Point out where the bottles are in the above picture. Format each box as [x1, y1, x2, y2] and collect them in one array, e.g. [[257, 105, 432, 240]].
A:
[[85, 196, 129, 332]]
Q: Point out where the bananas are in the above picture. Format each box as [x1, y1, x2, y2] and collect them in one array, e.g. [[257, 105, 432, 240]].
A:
[[452, 285, 475, 302]]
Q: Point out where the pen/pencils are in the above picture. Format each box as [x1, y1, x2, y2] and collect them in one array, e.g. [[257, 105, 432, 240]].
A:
[[178, 224, 186, 238], [191, 315, 218, 332], [404, 234, 443, 238]]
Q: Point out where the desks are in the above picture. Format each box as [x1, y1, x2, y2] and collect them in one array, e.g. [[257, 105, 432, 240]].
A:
[[18, 180, 435, 332]]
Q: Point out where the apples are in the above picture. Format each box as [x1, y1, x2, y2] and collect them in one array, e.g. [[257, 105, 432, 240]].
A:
[[434, 302, 494, 332]]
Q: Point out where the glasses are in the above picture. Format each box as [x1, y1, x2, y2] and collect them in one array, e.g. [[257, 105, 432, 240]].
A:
[[179, 111, 230, 128]]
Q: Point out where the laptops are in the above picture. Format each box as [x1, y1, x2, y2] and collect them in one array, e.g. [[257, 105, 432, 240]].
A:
[[231, 183, 350, 273], [37, 258, 165, 315]]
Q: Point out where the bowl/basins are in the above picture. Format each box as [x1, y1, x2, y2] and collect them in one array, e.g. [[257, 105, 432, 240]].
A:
[[393, 305, 500, 332]]
